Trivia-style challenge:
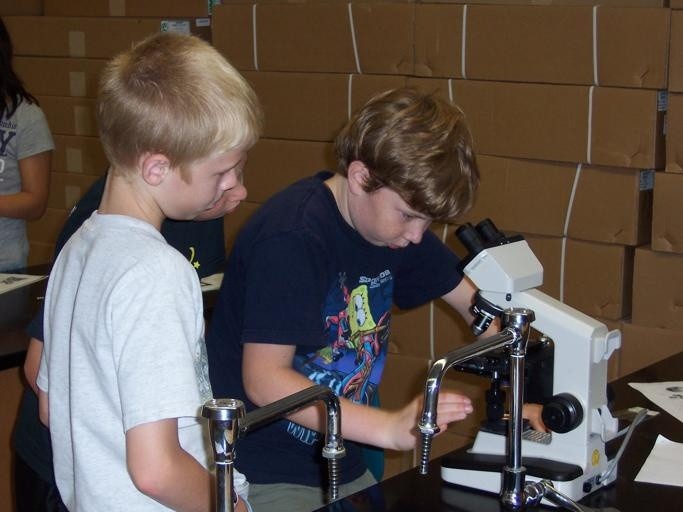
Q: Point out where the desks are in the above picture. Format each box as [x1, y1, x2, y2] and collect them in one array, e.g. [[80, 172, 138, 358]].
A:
[[313, 342, 681, 510]]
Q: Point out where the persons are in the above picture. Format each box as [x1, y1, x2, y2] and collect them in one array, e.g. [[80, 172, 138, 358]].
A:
[[10, 162, 256, 512], [217, 88, 550, 512], [33, 33, 267, 512], [0, 16, 57, 267]]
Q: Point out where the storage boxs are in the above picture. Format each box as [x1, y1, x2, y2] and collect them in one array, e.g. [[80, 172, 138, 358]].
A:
[[0, 0, 683, 473]]
